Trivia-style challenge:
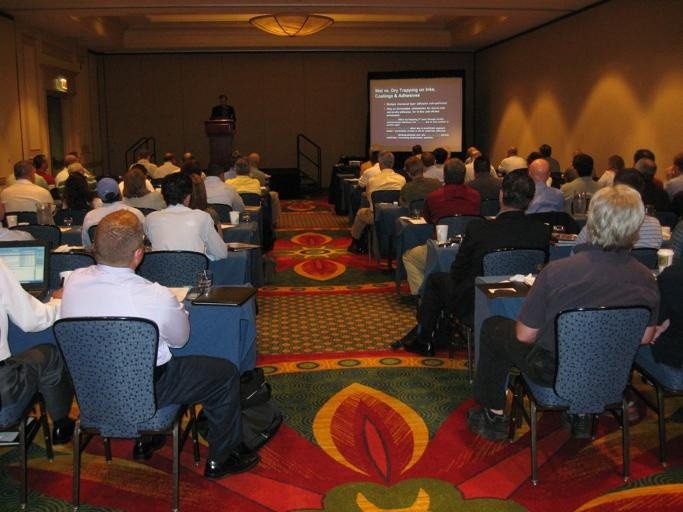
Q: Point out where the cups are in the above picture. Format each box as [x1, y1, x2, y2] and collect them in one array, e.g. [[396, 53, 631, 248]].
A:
[[64, 216, 73, 229], [414, 208, 421, 219], [242, 213, 250, 225], [6, 215, 17, 228], [657, 249, 674, 274], [662, 226, 671, 241], [645, 204, 656, 217], [229, 211, 240, 225], [435, 225, 448, 243], [59, 270, 73, 288], [197, 270, 212, 297]]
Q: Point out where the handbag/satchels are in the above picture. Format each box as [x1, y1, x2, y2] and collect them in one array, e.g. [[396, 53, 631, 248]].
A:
[[195, 366, 284, 453]]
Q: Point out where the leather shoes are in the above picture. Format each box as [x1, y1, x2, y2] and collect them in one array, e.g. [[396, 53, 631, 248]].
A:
[[203, 448, 260, 480], [347, 241, 361, 254], [134, 433, 167, 461], [406, 342, 436, 355], [52, 419, 75, 445]]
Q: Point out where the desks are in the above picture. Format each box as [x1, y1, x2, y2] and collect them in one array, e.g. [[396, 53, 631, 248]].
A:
[[238, 203, 264, 246], [471, 259, 674, 388], [384, 204, 588, 300], [336, 171, 358, 212], [411, 227, 579, 306], [344, 176, 358, 202], [54, 243, 265, 289], [0, 286, 257, 388], [57, 221, 260, 247], [374, 202, 403, 274], [352, 186, 364, 217]]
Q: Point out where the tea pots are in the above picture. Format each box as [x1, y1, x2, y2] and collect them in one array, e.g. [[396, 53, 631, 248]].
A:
[[35, 201, 58, 225], [573, 191, 586, 218]]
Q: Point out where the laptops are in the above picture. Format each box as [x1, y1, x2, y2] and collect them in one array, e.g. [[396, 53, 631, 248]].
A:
[[0, 241, 52, 299]]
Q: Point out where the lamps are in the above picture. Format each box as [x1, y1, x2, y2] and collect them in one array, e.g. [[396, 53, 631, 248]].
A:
[[55, 79, 71, 95], [249, 14, 333, 37]]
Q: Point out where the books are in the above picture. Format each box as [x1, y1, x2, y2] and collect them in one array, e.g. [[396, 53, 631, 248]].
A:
[[226, 242, 261, 252], [552, 233, 579, 247], [476, 280, 530, 300], [192, 288, 257, 306]]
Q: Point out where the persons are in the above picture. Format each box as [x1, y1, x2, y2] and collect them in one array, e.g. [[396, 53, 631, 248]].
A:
[[465, 184, 660, 442], [56, 155, 96, 185], [399, 156, 442, 216], [60, 211, 262, 479], [632, 149, 663, 190], [119, 163, 155, 196], [158, 153, 179, 179], [523, 158, 564, 214], [598, 155, 625, 187], [465, 147, 477, 164], [223, 150, 252, 183], [668, 219, 682, 260], [210, 94, 236, 132], [0, 161, 56, 224], [204, 165, 246, 218], [248, 152, 266, 188], [120, 169, 165, 211], [33, 154, 55, 188], [187, 174, 223, 238], [559, 153, 599, 213], [421, 152, 444, 183], [636, 263, 683, 373], [61, 172, 94, 212], [0, 196, 37, 242], [539, 144, 560, 174], [143, 172, 228, 263], [433, 147, 448, 170], [664, 150, 683, 202], [0, 258, 77, 446], [358, 151, 381, 188], [498, 146, 526, 177], [81, 178, 145, 247], [7, 159, 48, 190], [180, 152, 195, 169], [347, 151, 406, 254], [403, 144, 423, 169], [570, 169, 663, 265], [464, 151, 497, 183], [405, 170, 551, 355], [225, 158, 261, 200], [360, 145, 384, 177], [403, 159, 482, 305], [632, 158, 670, 214], [466, 156, 501, 200], [129, 150, 162, 178]]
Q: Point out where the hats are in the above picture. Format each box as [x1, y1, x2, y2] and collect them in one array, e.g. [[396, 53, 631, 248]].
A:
[[67, 163, 82, 174], [96, 177, 119, 202]]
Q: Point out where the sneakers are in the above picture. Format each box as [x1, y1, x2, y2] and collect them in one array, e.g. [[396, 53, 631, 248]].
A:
[[560, 408, 591, 440], [612, 393, 640, 426], [467, 408, 509, 437]]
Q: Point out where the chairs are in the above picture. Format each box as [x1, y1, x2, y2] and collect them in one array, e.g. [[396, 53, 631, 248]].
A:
[[56, 315, 202, 511], [6, 209, 40, 229], [361, 191, 403, 265], [505, 306, 650, 486], [599, 329, 683, 468], [480, 198, 503, 219], [577, 240, 659, 276], [11, 225, 61, 252], [133, 248, 211, 293], [436, 214, 485, 239], [527, 211, 577, 242], [44, 254, 98, 292], [0, 388, 56, 511], [239, 193, 259, 212], [406, 199, 427, 212], [86, 224, 100, 248], [132, 207, 160, 250], [436, 249, 546, 385], [49, 208, 94, 228], [205, 202, 233, 225]]
